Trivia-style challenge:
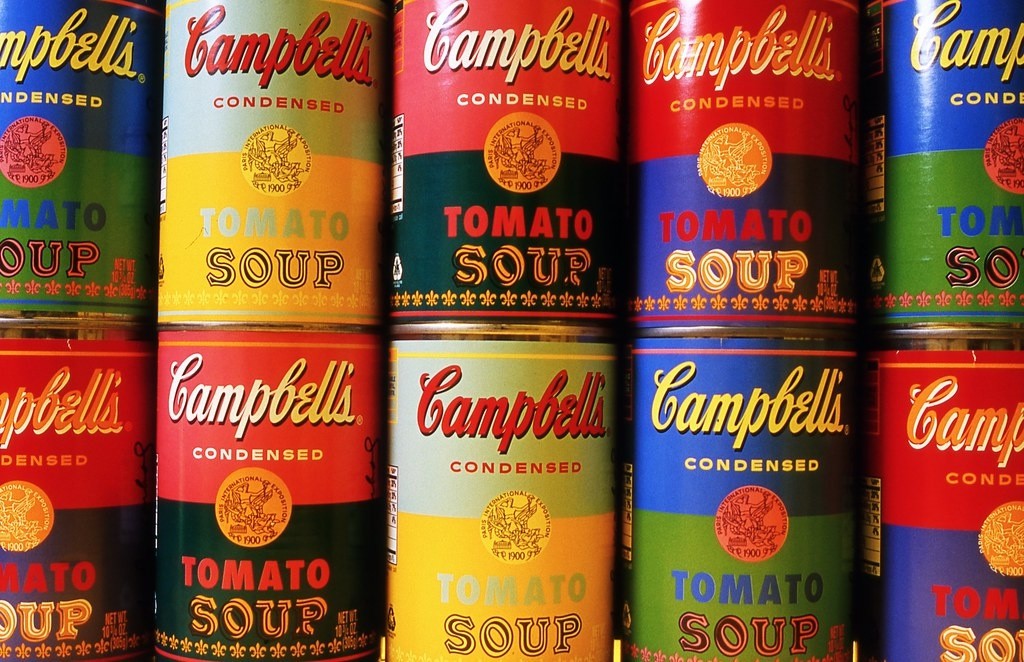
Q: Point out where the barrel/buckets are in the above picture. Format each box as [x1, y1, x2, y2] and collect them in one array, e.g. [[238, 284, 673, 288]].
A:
[[2, 0, 1024, 662]]
